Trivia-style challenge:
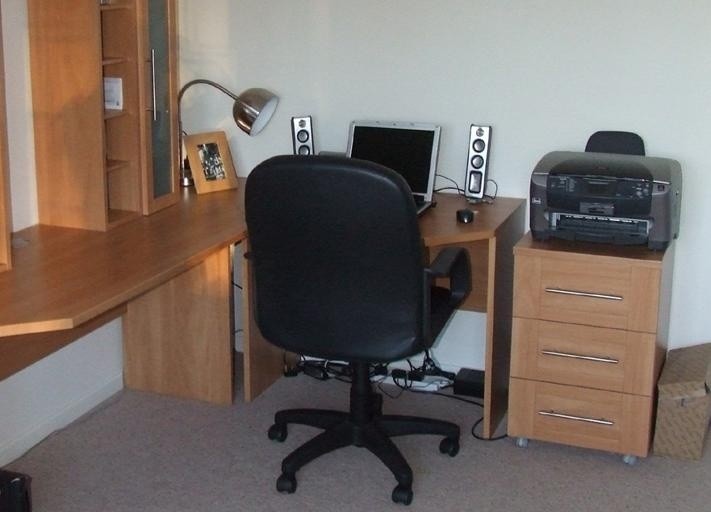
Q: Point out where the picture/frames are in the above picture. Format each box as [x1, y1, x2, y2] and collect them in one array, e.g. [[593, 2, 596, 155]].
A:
[[180, 130, 239, 195], [0, 22, 13, 277]]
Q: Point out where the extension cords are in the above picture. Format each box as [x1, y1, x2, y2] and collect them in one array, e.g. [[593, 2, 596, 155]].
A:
[[369, 367, 453, 392]]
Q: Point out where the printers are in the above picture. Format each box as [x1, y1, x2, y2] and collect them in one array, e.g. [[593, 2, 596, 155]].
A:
[[529, 151, 683, 252]]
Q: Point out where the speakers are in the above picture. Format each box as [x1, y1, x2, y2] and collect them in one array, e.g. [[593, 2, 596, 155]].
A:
[[463, 124, 492, 203], [291, 115, 315, 157]]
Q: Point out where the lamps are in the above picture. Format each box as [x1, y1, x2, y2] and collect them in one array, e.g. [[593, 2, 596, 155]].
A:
[[174, 77, 281, 190]]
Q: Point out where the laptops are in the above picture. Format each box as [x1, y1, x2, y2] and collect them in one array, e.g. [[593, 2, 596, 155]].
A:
[[345, 118, 441, 219]]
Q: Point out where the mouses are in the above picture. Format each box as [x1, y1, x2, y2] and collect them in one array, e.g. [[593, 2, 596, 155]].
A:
[[456, 209, 474, 224]]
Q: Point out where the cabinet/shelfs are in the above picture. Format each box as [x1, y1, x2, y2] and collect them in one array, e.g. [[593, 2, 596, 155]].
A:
[[29, 1, 184, 231], [504, 227, 678, 468]]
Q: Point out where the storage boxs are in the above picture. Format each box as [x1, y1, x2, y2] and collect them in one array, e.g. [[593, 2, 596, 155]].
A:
[[652, 342, 711, 462]]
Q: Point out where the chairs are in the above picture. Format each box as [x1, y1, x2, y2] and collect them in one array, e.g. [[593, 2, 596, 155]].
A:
[[242, 153, 473, 506]]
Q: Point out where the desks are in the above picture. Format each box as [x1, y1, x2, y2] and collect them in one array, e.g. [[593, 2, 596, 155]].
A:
[[0, 175, 247, 407], [242, 191, 529, 440]]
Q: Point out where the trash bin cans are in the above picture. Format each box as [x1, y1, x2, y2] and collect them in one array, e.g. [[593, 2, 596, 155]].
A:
[[0, 467, 32, 512]]
